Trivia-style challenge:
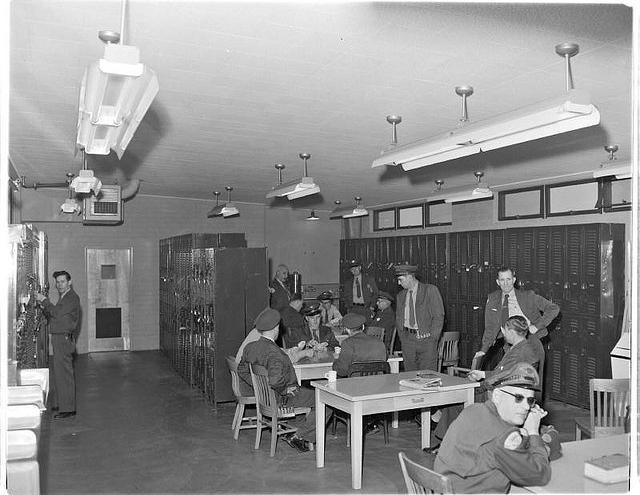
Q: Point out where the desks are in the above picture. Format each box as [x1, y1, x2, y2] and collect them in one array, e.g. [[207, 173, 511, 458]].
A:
[[310, 370, 481, 490], [508, 433, 630, 495]]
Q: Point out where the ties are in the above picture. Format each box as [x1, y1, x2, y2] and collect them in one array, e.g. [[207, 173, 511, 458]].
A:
[[501, 295, 509, 323]]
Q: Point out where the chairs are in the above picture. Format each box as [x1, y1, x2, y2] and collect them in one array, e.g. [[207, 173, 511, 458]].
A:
[[224, 356, 283, 441], [248, 362, 314, 457], [575, 378, 631, 440], [398, 450, 453, 494]]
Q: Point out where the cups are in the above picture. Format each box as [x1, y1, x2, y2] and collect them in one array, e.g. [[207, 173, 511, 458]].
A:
[[334, 347, 341, 355], [325, 371, 337, 385]]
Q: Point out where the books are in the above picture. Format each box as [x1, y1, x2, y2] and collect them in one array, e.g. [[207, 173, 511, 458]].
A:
[[583, 453, 629, 485], [398, 372, 443, 391]]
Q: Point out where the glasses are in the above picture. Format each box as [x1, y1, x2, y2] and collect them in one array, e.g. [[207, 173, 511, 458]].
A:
[[500, 388, 538, 407]]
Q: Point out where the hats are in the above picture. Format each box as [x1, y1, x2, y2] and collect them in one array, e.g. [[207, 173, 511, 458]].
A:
[[485, 362, 540, 392], [254, 259, 420, 331]]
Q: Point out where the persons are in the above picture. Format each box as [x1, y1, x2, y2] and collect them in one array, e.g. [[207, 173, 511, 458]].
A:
[[416, 315, 541, 454], [432, 361, 563, 494], [238, 308, 335, 455], [229, 309, 315, 397], [473, 264, 561, 361], [391, 263, 444, 415], [33, 270, 82, 420], [332, 312, 387, 439], [230, 256, 395, 346]]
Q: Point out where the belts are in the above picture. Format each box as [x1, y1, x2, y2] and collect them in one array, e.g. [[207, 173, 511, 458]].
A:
[[404, 328, 416, 334]]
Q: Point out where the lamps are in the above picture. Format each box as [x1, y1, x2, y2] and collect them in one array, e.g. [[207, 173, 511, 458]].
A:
[[329, 195, 369, 219], [371, 42, 601, 172], [303, 210, 322, 221], [265, 153, 321, 200], [61, 0, 161, 214], [206, 186, 240, 218], [426, 172, 494, 203], [592, 145, 632, 178]]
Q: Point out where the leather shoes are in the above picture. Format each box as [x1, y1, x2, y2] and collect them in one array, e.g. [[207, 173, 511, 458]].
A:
[[52, 406, 75, 419], [423, 444, 440, 456], [286, 434, 309, 452], [414, 414, 436, 427], [364, 422, 379, 434]]
